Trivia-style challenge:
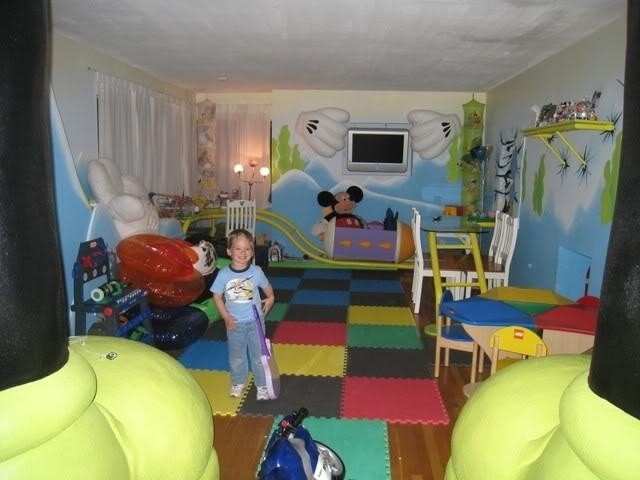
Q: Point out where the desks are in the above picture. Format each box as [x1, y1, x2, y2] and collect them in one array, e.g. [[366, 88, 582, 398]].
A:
[[440, 296, 537, 383], [534, 305, 598, 356], [421, 214, 499, 336]]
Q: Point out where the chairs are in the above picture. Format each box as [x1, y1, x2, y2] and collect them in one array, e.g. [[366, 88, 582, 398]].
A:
[[462, 325, 548, 400], [224, 199, 258, 267], [411, 207, 465, 316], [465, 215, 520, 297], [412, 209, 459, 302], [577, 296, 600, 312], [466, 210, 510, 293], [435, 287, 485, 386]]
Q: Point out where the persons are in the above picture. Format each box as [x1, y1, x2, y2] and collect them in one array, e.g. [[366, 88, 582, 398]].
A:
[[208, 229, 279, 402]]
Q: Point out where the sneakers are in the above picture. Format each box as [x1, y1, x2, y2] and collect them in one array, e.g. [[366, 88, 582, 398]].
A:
[[256, 386, 269, 401], [230, 383, 245, 398]]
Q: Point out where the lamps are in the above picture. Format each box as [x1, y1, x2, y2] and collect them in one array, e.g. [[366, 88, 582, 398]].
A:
[[233, 159, 270, 201]]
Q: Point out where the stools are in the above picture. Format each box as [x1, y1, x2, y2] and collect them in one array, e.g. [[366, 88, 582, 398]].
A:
[[482, 284, 560, 314]]
